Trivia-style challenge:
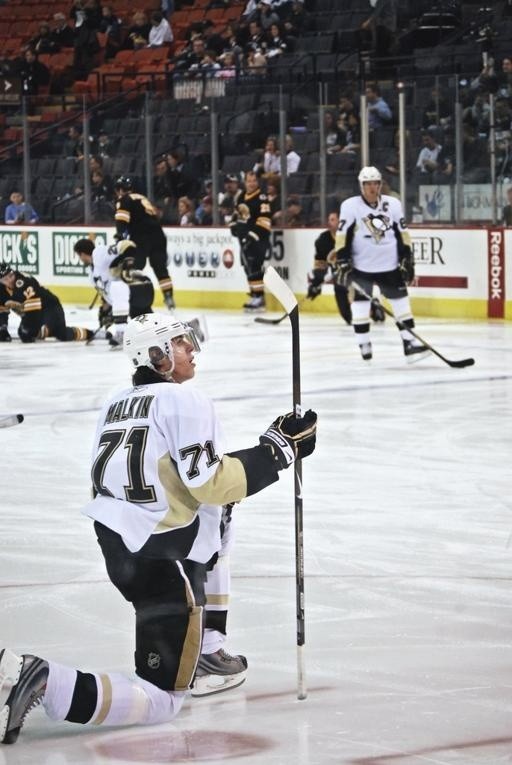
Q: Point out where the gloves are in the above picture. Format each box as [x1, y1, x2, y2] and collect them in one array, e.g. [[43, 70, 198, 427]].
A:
[[258, 409, 317, 468], [399, 256, 415, 283], [335, 259, 357, 287], [307, 282, 322, 297]]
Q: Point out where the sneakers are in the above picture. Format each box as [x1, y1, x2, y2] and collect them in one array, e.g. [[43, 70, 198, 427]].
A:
[[0, 654, 48, 744], [166, 297, 174, 307], [196, 647, 247, 676], [243, 295, 266, 309], [92, 330, 125, 346], [360, 342, 372, 359], [402, 336, 429, 356]]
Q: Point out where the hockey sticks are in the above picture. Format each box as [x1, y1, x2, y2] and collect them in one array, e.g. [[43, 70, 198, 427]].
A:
[[348, 278, 475, 368], [262, 266, 307, 700], [253, 296, 311, 326]]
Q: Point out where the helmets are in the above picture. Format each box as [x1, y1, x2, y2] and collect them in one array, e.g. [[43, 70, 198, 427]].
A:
[[1, 262, 13, 278], [111, 175, 136, 191], [123, 310, 194, 376], [357, 165, 385, 197]]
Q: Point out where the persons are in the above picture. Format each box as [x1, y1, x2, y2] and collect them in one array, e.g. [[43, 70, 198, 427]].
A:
[[305, 210, 385, 325], [113, 174, 176, 310], [0, 310, 317, 743], [0, 262, 107, 344], [336, 167, 429, 360], [109, 240, 153, 320], [1, 0, 511, 229], [230, 169, 272, 309], [74, 240, 131, 351]]
